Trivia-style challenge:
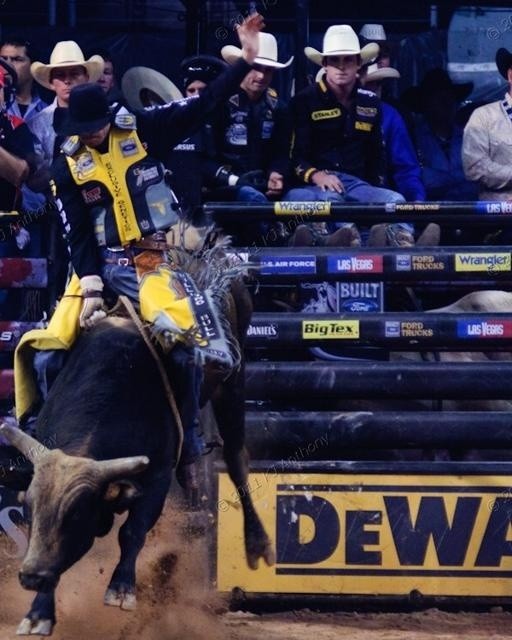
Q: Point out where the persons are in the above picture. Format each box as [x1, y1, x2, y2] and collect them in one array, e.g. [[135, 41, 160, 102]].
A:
[[0, 10, 512, 490], [1, 11, 512, 259]]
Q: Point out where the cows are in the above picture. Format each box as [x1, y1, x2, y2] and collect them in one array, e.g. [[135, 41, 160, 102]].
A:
[[0, 241, 275, 637], [387, 291, 512, 461]]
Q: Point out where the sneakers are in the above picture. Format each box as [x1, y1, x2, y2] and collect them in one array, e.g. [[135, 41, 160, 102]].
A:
[[284, 222, 441, 247], [176, 463, 200, 490]]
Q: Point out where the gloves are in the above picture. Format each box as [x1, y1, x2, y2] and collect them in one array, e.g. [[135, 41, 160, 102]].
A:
[[226, 168, 267, 193], [79, 275, 107, 328]]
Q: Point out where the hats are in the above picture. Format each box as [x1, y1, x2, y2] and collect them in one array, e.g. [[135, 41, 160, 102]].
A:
[[304, 24, 400, 87], [31, 30, 295, 135], [0, 64, 9, 88], [495, 47, 512, 80], [400, 68, 474, 113]]
